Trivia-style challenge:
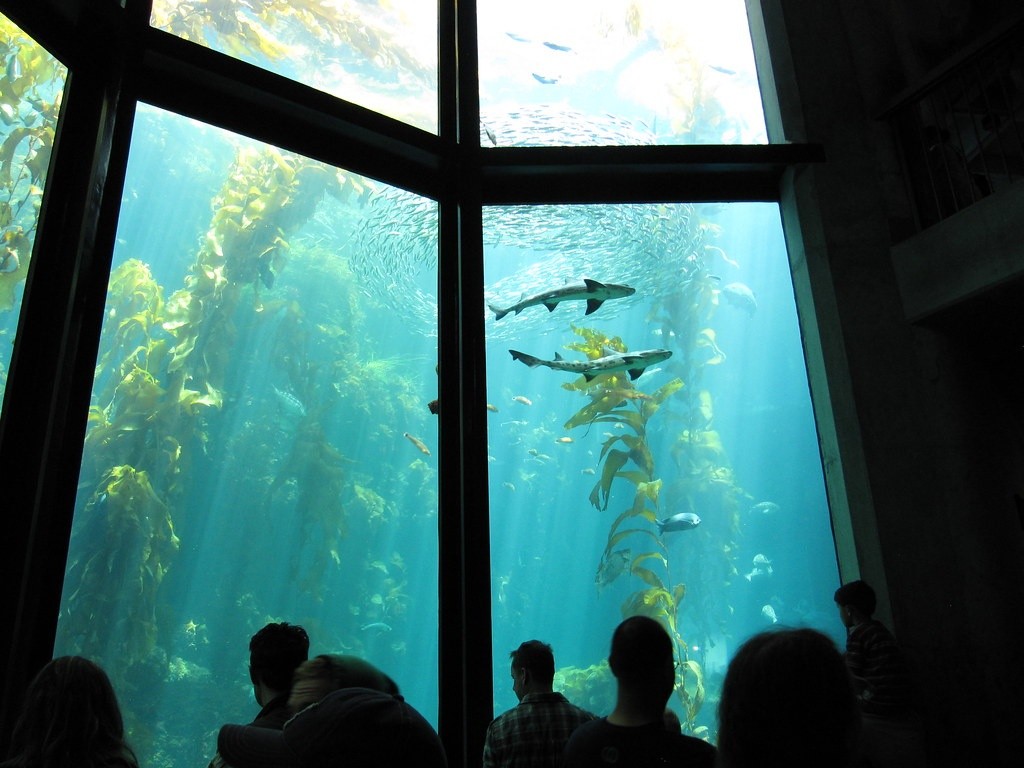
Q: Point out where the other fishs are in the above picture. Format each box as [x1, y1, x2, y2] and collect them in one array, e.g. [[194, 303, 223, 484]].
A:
[[349, 108, 713, 333], [361, 622, 394, 638], [507, 30, 571, 85], [655, 513, 701, 536], [723, 285, 759, 319], [487, 394, 596, 490], [404, 432, 433, 457]]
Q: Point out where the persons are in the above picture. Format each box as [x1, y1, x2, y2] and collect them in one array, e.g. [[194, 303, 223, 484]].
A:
[[285, 654, 404, 719], [205, 620, 311, 768], [835, 581, 923, 768], [716, 623, 856, 768], [563, 615, 720, 768], [1, 655, 139, 768], [482, 639, 600, 768], [217, 687, 451, 768]]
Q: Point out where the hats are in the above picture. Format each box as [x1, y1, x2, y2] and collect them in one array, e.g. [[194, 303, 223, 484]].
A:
[[218, 686, 447, 768], [288, 656, 399, 706]]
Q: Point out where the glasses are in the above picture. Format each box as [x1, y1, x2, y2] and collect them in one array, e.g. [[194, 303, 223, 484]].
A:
[[673, 660, 679, 669]]
